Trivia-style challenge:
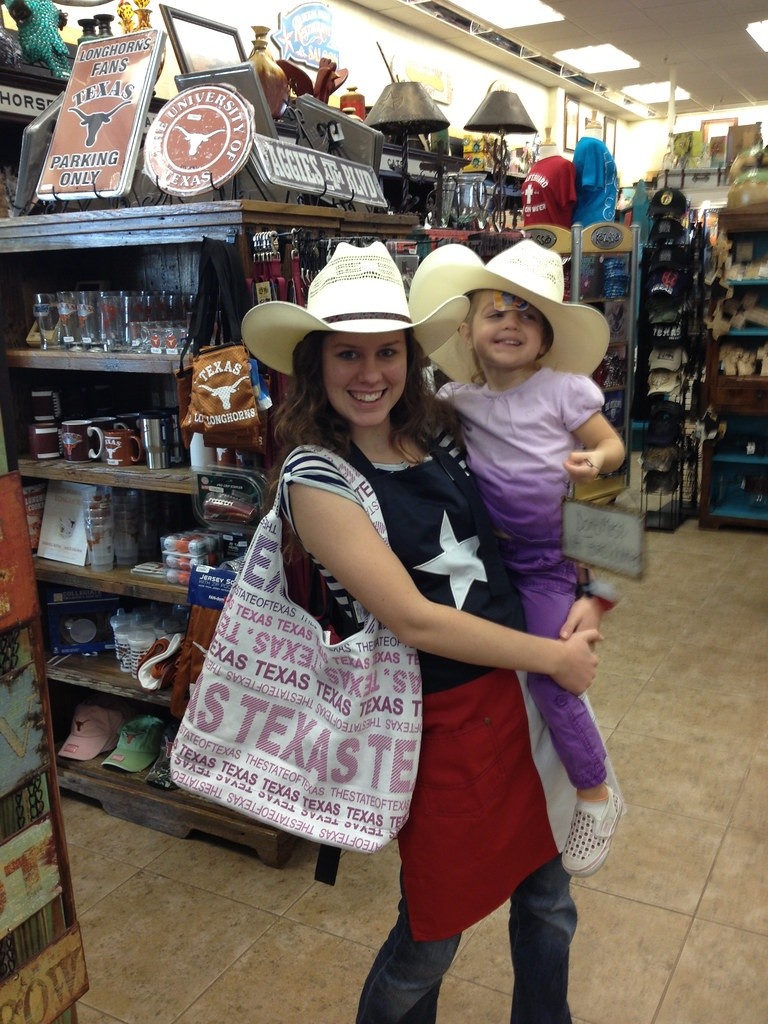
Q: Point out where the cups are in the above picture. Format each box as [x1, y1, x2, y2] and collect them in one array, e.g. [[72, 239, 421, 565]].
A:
[[110, 602, 187, 679], [31, 291, 221, 354], [82, 487, 184, 571], [28, 386, 141, 466]]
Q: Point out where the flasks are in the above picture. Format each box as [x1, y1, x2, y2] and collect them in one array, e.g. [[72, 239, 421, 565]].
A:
[[139, 406, 183, 473]]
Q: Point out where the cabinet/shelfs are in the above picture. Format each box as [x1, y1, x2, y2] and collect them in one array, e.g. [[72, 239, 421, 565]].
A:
[[0, 82, 768, 871]]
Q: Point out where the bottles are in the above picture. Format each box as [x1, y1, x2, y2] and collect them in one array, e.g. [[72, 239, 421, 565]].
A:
[[118, 0, 166, 97], [247, 27, 288, 119], [339, 87, 365, 122]]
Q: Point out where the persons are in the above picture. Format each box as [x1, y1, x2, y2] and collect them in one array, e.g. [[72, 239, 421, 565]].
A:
[[572, 129, 617, 229], [242, 241, 606, 1024], [409, 239, 625, 878], [521, 145, 577, 230]]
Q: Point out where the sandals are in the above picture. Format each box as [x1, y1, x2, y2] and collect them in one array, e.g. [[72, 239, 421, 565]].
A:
[[559, 787, 623, 873]]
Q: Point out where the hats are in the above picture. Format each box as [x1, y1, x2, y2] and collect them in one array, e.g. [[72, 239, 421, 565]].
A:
[[57, 703, 130, 760], [102, 714, 162, 772], [238, 240, 476, 383], [407, 240, 611, 387], [642, 188, 685, 492], [147, 738, 180, 792]]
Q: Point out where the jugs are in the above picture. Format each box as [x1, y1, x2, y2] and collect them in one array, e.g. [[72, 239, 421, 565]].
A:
[[426, 174, 487, 229]]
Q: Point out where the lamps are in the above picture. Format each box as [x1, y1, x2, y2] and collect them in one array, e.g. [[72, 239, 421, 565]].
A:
[[464, 92, 539, 234], [363, 81, 449, 228]]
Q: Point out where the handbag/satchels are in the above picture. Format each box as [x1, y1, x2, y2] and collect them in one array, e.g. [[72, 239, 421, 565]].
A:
[[178, 236, 264, 447], [166, 444, 419, 850]]
